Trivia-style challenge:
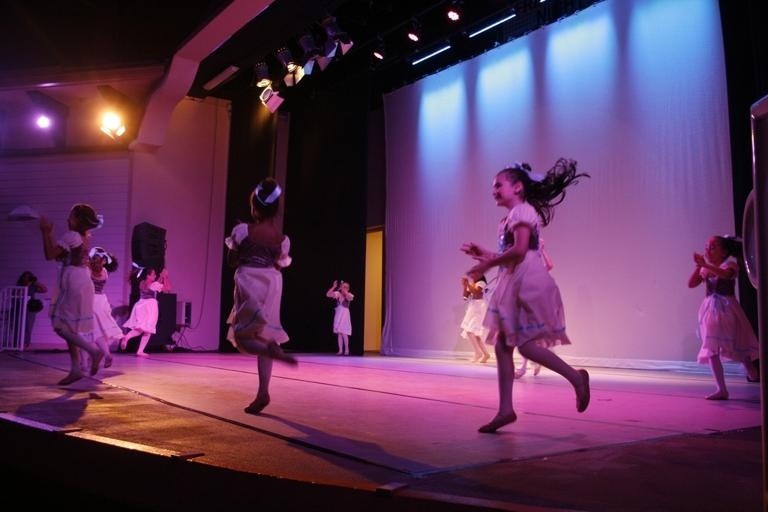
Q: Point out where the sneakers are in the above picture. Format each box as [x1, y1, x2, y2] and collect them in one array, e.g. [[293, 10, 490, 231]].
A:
[[244, 393, 270, 412], [474, 353, 589, 431], [267, 340, 297, 365], [57, 337, 149, 387], [704, 390, 729, 399]]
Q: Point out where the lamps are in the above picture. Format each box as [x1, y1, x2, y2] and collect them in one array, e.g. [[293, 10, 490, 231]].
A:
[[251, 14, 354, 115], [370, 0, 465, 64]]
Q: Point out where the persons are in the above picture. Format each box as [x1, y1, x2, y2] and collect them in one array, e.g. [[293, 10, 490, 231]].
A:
[[10, 269, 48, 351], [457, 157, 592, 433], [122, 263, 169, 359], [462, 272, 491, 365], [88, 247, 126, 371], [513, 236, 552, 381], [226, 178, 300, 414], [687, 234, 760, 402], [327, 279, 355, 358], [35, 201, 103, 386]]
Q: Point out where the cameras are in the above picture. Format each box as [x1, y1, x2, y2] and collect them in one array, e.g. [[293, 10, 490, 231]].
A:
[[29, 275, 37, 282]]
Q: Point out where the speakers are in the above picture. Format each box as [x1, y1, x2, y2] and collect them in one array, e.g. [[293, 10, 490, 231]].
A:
[[131, 222, 167, 273], [129, 293, 176, 353], [176, 301, 191, 325]]
